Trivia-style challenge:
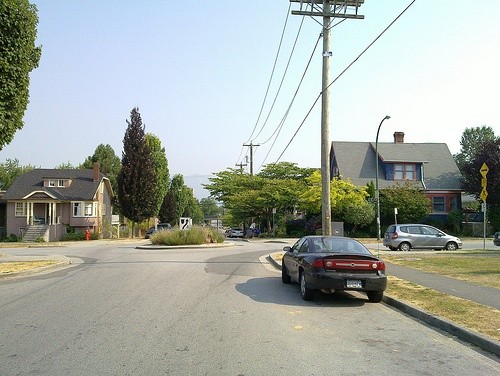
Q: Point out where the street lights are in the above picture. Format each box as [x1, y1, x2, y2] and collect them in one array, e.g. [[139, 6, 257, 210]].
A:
[[374, 115, 392, 241]]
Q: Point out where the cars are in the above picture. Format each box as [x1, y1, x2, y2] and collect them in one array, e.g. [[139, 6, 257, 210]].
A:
[[280, 235, 387, 303]]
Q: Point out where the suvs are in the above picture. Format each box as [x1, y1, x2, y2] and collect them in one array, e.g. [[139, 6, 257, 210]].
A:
[[144, 223, 171, 239], [383, 223, 463, 251], [230, 227, 243, 238]]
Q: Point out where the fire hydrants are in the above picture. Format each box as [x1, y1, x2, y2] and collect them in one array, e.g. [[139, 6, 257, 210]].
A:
[[85, 229, 90, 240]]
